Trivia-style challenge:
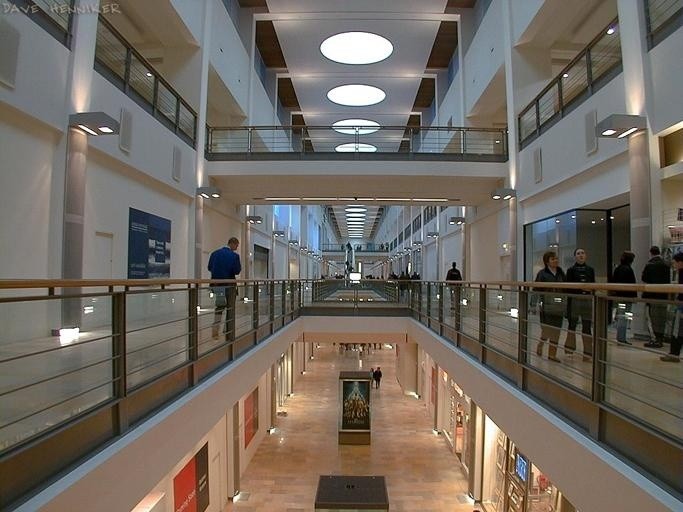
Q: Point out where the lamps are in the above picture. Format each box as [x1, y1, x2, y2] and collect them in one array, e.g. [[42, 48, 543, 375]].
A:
[[491, 189, 516, 201], [69, 111, 120, 136], [594, 113, 646, 140], [370, 217, 466, 271], [245, 214, 333, 267], [197, 187, 222, 199]]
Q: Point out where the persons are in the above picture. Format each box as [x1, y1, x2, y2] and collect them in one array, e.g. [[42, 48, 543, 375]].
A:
[[641, 246, 668, 348], [660, 253, 683, 362], [613, 251, 636, 346], [368, 368, 374, 390], [327, 270, 423, 298], [529, 252, 565, 362], [445, 262, 462, 310], [566, 248, 595, 363], [374, 367, 381, 389], [207, 238, 241, 340]]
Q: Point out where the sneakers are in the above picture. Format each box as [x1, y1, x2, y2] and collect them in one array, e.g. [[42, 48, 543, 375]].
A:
[[661, 355, 679, 362], [644, 340, 660, 348]]
